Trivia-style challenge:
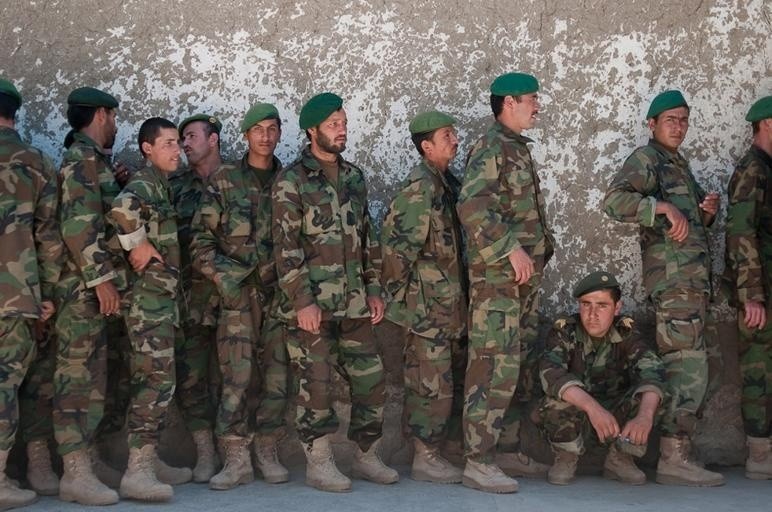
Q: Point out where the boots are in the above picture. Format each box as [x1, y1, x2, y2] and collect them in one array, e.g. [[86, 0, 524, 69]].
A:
[[349, 433, 401, 485], [151, 448, 194, 485], [547, 442, 580, 486], [743, 431, 772, 483], [24, 437, 60, 497], [442, 438, 469, 466], [250, 434, 292, 485], [209, 433, 255, 491], [57, 446, 120, 507], [187, 425, 219, 484], [462, 457, 519, 495], [654, 433, 726, 488], [89, 442, 116, 486], [214, 437, 225, 467], [119, 443, 172, 502], [0, 445, 38, 511], [495, 449, 553, 481], [602, 441, 648, 487], [299, 432, 354, 493], [409, 435, 465, 484]]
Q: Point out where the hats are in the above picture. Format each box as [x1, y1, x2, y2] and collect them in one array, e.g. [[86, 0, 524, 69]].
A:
[[745, 95, 772, 123], [0, 78, 23, 108], [489, 72, 539, 95], [238, 102, 281, 137], [66, 85, 120, 111], [409, 110, 458, 135], [645, 90, 686, 122], [298, 91, 344, 130], [62, 129, 80, 150], [177, 113, 223, 134], [573, 270, 623, 298]]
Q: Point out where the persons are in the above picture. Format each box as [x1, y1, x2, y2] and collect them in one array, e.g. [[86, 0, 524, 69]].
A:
[[380, 111, 468, 486], [603, 89, 725, 487], [1, 78, 64, 507], [50, 87, 121, 508], [168, 113, 242, 481], [108, 118, 192, 501], [453, 73, 554, 494], [271, 93, 400, 491], [188, 102, 290, 491], [721, 94, 772, 482], [530, 271, 665, 486]]
